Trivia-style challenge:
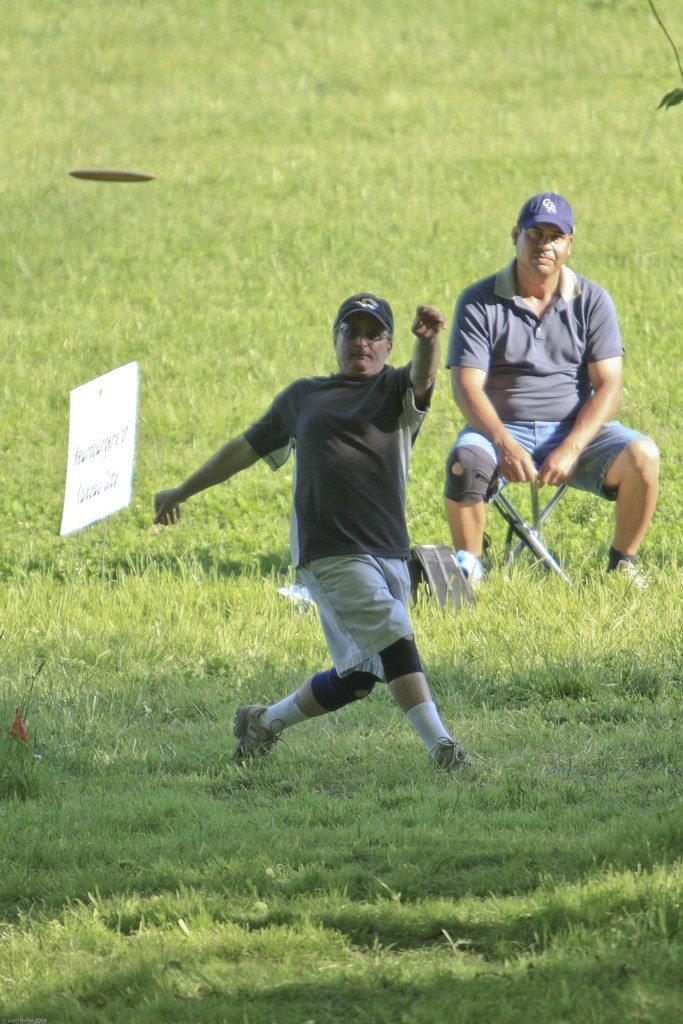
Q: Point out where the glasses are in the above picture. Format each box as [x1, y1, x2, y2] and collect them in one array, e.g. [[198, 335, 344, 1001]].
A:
[[335, 326, 390, 341]]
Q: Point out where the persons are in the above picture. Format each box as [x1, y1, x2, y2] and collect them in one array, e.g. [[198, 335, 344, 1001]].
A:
[[153, 293, 490, 781], [443, 192, 660, 594]]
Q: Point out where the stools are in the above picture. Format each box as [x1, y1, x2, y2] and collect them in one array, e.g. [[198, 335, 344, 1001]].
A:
[[491, 481, 571, 581]]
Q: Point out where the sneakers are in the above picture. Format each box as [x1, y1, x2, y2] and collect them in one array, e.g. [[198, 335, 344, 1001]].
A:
[[428, 735, 472, 774], [453, 550, 483, 582], [231, 705, 294, 764], [606, 560, 650, 590]]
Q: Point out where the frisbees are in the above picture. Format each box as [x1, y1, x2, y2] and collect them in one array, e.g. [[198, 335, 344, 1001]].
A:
[[70, 170, 156, 182]]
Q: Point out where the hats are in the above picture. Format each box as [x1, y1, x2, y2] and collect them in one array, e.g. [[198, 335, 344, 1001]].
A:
[[516, 192, 573, 235], [334, 293, 394, 339]]
